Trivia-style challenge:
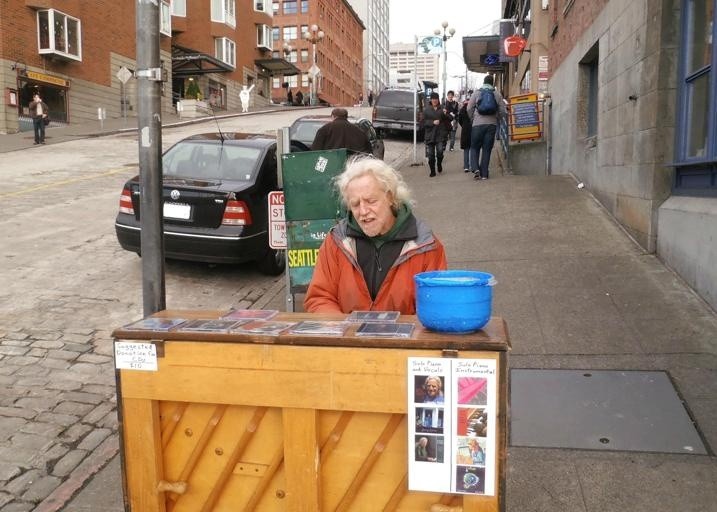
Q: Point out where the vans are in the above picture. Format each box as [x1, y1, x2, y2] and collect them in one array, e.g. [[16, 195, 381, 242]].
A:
[[371, 87, 424, 143]]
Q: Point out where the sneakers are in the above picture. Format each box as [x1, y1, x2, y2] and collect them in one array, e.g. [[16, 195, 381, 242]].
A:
[[473, 170, 480, 179], [482, 176, 487, 180], [464, 168, 469, 173]]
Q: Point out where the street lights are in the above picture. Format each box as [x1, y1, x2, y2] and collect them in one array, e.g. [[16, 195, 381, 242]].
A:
[[434, 19, 456, 109], [304, 26, 325, 106]]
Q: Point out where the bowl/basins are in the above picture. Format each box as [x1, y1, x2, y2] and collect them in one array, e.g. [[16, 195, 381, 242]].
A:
[[412, 269, 499, 335]]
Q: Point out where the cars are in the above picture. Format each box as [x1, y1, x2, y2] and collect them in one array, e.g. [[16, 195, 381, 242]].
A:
[[115, 133, 312, 276], [289, 115, 384, 160]]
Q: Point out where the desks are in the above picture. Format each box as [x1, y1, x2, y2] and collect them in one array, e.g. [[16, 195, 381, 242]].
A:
[[109, 309, 512, 510]]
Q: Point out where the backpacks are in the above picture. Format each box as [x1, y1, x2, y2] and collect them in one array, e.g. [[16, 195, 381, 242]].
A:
[[477, 88, 498, 116]]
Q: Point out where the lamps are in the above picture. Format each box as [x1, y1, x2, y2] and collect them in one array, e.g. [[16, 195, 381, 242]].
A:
[[10, 58, 29, 82]]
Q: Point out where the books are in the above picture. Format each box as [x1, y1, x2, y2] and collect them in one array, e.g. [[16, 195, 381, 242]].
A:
[[120, 306, 417, 340]]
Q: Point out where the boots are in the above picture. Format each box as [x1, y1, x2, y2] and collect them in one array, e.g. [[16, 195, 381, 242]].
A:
[[430, 163, 436, 177], [437, 160, 442, 173]]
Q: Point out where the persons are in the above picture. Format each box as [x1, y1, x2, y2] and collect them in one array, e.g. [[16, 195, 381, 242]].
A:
[[295, 90, 304, 105], [311, 109, 373, 157], [426, 414, 432, 432], [469, 88, 473, 94], [466, 76, 507, 182], [421, 92, 446, 177], [28, 94, 49, 144], [415, 436, 430, 461], [239, 84, 255, 112], [467, 440, 484, 465], [458, 98, 473, 172], [286, 89, 294, 105], [359, 92, 364, 104], [421, 376, 444, 403], [442, 90, 458, 151], [303, 155, 447, 315], [368, 93, 373, 107]]
